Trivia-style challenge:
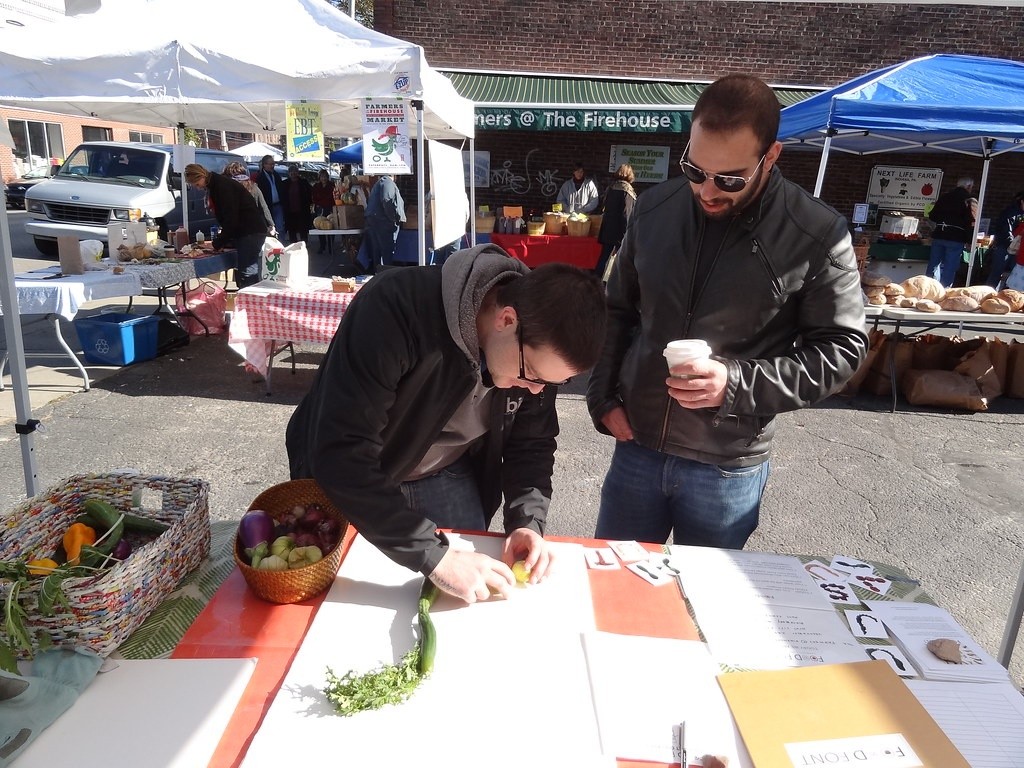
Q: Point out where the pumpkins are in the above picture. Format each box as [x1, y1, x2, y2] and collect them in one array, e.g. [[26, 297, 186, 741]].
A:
[[313, 208, 334, 229]]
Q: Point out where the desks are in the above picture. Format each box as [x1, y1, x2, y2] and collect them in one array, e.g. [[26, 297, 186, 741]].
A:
[[0, 270, 141, 396], [225, 276, 367, 399], [870, 302, 1024, 414], [492, 231, 603, 270], [358, 229, 492, 269], [110, 521, 1024, 768], [956, 248, 984, 287], [27, 248, 238, 338], [308, 228, 362, 276], [861, 306, 884, 330]]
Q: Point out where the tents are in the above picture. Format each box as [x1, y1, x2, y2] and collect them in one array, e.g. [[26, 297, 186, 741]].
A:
[[0, 0, 476, 498], [776, 54, 1024, 339], [329, 140, 363, 181]]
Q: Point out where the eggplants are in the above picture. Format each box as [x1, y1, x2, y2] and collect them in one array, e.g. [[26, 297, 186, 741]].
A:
[[104, 538, 132, 565], [240, 510, 275, 570]]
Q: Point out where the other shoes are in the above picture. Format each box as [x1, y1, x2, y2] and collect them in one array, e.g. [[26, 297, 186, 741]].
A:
[[328, 245, 332, 254], [318, 247, 325, 254]]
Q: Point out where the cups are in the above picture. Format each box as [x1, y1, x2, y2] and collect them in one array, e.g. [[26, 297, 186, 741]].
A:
[[164, 245, 174, 258], [663, 339, 712, 379], [167, 232, 176, 246]]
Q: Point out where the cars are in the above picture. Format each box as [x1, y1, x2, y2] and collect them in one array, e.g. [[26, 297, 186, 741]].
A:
[[4, 164, 91, 210], [247, 164, 290, 187]]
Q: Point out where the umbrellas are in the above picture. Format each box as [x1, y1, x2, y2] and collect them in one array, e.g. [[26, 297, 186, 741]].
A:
[[228, 142, 284, 163]]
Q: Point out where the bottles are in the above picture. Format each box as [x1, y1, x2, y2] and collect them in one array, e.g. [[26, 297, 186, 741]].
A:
[[196, 230, 204, 244], [210, 225, 218, 240], [175, 226, 188, 253]]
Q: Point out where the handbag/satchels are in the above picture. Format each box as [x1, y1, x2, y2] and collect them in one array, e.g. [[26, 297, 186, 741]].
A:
[[1007, 235, 1022, 256], [175, 276, 227, 335], [260, 237, 308, 288]]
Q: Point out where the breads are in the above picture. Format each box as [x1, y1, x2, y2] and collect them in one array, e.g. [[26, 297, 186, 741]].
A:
[[860, 272, 1024, 315]]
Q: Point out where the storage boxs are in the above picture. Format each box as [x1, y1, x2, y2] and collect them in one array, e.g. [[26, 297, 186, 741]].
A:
[[332, 280, 356, 293], [226, 293, 237, 311], [880, 216, 919, 236], [73, 311, 163, 368], [402, 203, 432, 230], [332, 204, 364, 229]]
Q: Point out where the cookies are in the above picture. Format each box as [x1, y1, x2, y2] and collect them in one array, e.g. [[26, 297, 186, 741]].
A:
[[927, 639, 962, 664]]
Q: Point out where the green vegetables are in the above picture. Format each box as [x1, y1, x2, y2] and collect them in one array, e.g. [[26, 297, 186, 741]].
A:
[[323, 637, 425, 717], [0, 542, 130, 675]]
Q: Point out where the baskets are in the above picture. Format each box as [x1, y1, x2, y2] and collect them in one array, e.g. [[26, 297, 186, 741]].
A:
[[852, 237, 870, 259], [234, 478, 351, 604], [0, 472, 208, 659]]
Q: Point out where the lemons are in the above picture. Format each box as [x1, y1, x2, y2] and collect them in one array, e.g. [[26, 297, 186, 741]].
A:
[[511, 561, 532, 582]]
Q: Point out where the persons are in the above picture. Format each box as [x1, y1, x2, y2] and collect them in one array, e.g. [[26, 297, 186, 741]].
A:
[[255, 155, 334, 245], [340, 169, 367, 262], [556, 163, 598, 214], [925, 177, 978, 288], [220, 161, 276, 283], [594, 164, 638, 278], [365, 175, 407, 273], [185, 164, 268, 288], [283, 243, 606, 603], [587, 76, 868, 549], [985, 190, 1024, 292]]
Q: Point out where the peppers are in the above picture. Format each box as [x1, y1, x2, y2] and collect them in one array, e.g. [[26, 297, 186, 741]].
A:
[[26, 558, 60, 575], [63, 522, 96, 565]]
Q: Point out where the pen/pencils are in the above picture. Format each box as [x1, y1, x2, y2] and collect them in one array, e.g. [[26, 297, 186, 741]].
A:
[[681, 719, 687, 766]]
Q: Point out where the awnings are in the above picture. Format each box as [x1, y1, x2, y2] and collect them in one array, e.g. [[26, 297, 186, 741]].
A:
[[429, 67, 833, 133]]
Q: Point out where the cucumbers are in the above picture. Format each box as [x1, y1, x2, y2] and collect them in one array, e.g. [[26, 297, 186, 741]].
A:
[[76, 500, 173, 552]]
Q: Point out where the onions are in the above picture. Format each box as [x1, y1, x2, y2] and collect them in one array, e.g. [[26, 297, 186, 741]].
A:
[[281, 507, 338, 553]]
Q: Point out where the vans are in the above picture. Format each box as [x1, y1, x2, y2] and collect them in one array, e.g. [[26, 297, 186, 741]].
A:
[[23, 142, 252, 254]]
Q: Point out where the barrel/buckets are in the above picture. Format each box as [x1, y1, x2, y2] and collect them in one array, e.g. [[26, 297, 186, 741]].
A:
[[528, 212, 603, 237], [80, 240, 104, 264], [476, 210, 496, 234]]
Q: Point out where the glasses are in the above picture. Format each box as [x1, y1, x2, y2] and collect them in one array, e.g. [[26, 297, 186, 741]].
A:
[[267, 164, 275, 167], [679, 138, 766, 193], [503, 303, 571, 387]]
